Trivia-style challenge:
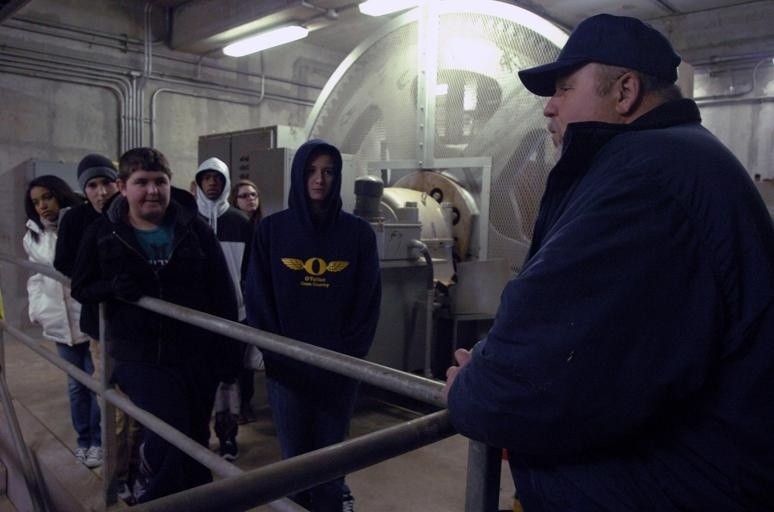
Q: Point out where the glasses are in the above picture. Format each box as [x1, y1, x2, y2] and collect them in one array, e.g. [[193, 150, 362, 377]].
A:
[[237, 192, 259, 199]]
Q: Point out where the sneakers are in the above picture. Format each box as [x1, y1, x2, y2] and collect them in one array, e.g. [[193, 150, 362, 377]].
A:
[[75, 444, 105, 468], [218, 437, 241, 461]]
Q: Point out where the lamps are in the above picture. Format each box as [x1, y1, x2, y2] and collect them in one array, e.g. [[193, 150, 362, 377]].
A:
[[222, 22, 309, 58], [356, 0, 420, 17]]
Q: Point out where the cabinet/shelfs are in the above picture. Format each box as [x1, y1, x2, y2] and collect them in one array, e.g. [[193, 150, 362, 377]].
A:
[[194, 125, 359, 219]]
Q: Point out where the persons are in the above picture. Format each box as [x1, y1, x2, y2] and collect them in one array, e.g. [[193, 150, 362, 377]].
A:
[[193, 157, 254, 461], [70, 146, 239, 503], [439, 11, 774, 511], [52, 152, 148, 505], [226, 180, 265, 428], [239, 138, 383, 511], [20, 173, 102, 469]]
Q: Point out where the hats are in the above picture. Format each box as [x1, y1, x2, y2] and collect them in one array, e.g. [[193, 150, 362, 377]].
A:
[[518, 14, 681, 96], [77, 154, 119, 197]]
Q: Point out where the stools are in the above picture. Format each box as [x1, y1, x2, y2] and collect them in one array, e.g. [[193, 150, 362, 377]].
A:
[[451, 314, 496, 365]]
